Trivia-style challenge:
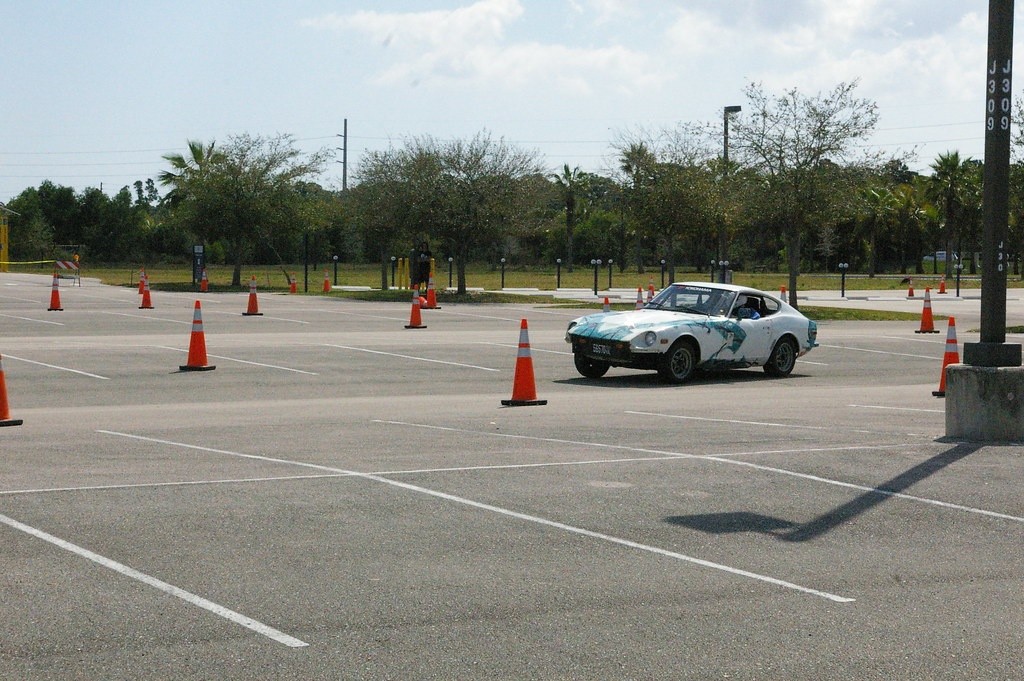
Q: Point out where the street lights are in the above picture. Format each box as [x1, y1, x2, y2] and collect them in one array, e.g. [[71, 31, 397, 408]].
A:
[[717, 105, 742, 284]]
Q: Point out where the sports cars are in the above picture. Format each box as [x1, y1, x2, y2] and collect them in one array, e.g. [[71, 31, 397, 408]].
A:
[[564, 282, 820, 387]]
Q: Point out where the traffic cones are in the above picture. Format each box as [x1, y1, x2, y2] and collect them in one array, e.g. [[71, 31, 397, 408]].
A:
[[781, 284, 787, 304], [137, 266, 145, 294], [418, 296, 427, 309], [914, 286, 940, 332], [196, 269, 209, 293], [0, 354, 24, 426], [242, 275, 264, 315], [48, 273, 63, 312], [646, 275, 655, 303], [602, 297, 610, 313], [289, 271, 297, 293], [500, 318, 548, 405], [907, 275, 915, 297], [422, 271, 441, 309], [405, 285, 427, 329], [179, 300, 217, 371], [937, 273, 948, 295], [636, 287, 645, 310], [932, 316, 959, 396], [323, 268, 331, 292], [139, 276, 154, 309]]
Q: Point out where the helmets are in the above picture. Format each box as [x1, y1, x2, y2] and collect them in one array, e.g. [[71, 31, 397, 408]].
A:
[[725, 292, 748, 309]]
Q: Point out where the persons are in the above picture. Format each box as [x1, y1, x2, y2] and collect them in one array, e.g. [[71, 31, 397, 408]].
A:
[[409, 242, 432, 291], [733, 303, 760, 319]]
